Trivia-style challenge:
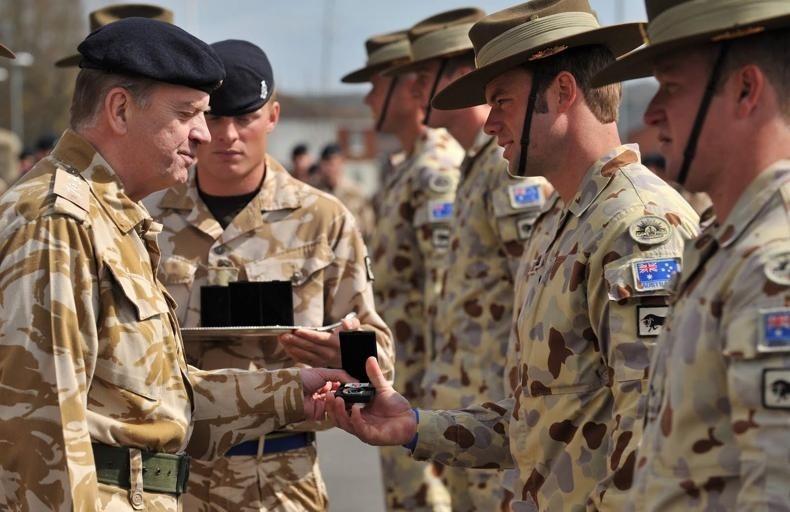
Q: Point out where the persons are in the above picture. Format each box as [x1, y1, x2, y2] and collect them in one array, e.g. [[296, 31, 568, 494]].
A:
[[18, 133, 60, 173], [340, 28, 464, 512], [591, 0, 789, 512], [137, 39, 396, 512], [380, 7, 554, 512], [325, 0, 700, 511], [1, 15, 360, 511], [640, 152, 667, 179], [288, 140, 376, 244]]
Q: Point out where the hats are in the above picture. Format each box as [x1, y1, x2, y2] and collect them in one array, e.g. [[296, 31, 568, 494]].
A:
[[431, 0, 648, 110], [340, 30, 428, 83], [207, 38, 274, 115], [382, 7, 486, 76], [53, 5, 174, 67], [76, 17, 226, 95], [589, 0, 790, 89]]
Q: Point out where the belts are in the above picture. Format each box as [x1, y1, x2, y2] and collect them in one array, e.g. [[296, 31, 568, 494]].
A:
[[226, 429, 315, 456], [90, 440, 191, 497]]
